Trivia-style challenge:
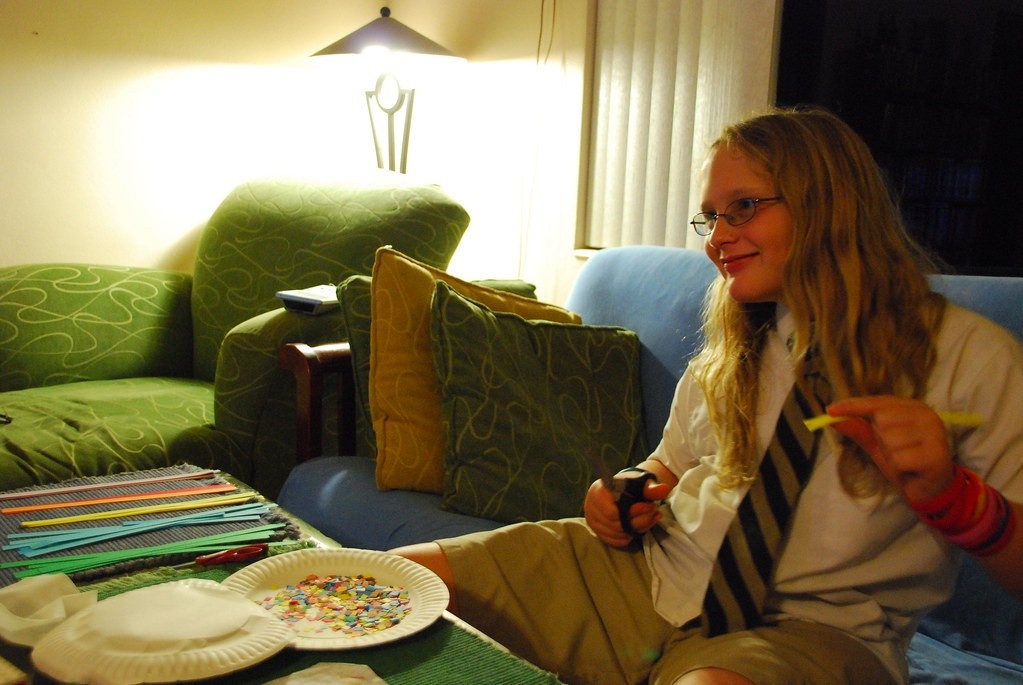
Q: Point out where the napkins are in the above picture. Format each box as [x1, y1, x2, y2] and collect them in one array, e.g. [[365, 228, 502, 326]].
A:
[[0, 571, 100, 648]]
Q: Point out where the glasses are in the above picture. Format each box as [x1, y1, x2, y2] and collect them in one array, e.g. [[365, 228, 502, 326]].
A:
[[690, 196, 785, 236]]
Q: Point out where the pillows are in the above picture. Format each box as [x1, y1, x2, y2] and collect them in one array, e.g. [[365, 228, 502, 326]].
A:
[[367, 244, 582, 492], [335, 277, 539, 462], [426, 280, 648, 522]]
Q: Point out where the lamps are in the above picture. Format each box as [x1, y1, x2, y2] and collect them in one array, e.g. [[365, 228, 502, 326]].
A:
[[310, 7, 469, 174]]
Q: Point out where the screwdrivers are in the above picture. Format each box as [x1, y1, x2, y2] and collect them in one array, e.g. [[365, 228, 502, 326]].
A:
[[173, 543, 271, 570]]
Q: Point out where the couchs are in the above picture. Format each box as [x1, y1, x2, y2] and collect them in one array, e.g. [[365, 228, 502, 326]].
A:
[[1, 176, 539, 491], [278, 244, 1022, 684]]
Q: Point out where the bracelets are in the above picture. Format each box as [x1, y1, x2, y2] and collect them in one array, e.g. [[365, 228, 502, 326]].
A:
[[615, 466, 661, 484], [903, 462, 1015, 558]]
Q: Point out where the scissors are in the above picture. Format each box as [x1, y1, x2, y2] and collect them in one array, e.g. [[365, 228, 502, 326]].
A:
[[581, 444, 661, 541]]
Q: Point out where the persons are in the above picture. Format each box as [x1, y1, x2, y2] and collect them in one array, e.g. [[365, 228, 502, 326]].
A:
[[384, 108, 1023, 684]]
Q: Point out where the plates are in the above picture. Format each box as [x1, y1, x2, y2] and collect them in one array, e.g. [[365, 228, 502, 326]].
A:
[[29, 577, 290, 685], [218, 548, 450, 650]]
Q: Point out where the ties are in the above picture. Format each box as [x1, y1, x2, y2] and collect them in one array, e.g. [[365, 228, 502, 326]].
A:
[[702, 334, 835, 639]]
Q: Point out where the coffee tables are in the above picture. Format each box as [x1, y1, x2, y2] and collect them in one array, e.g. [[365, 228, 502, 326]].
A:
[[1, 461, 564, 684]]
